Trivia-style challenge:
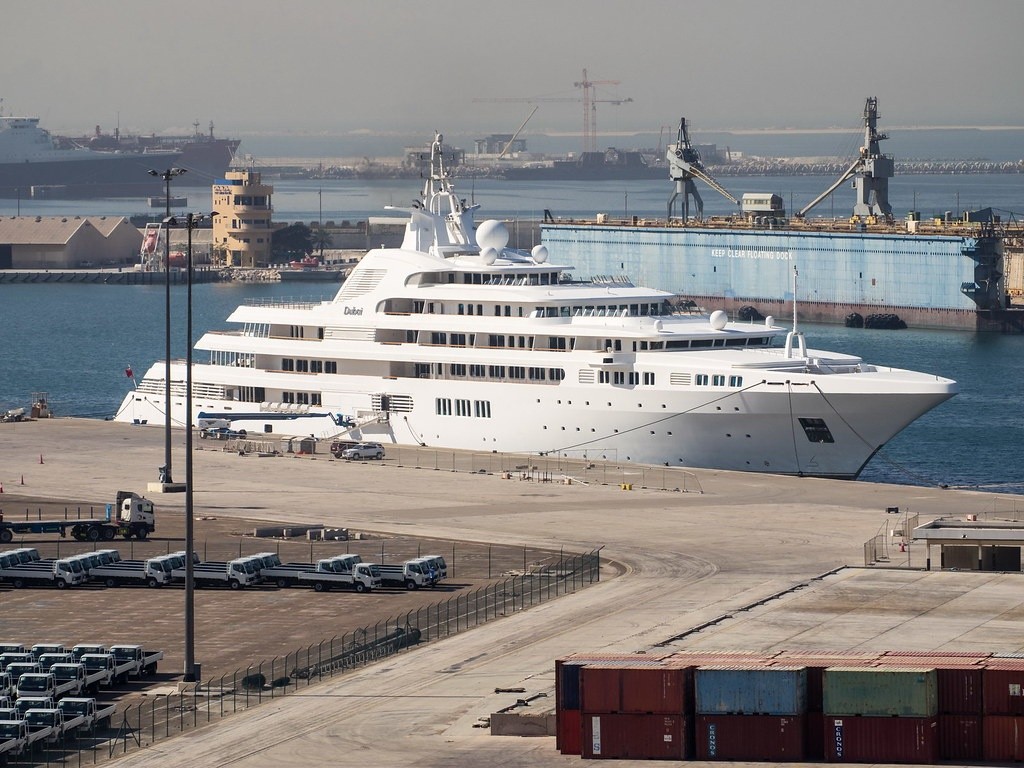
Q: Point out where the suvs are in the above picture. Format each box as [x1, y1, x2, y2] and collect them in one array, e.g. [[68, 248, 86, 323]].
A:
[[331, 441, 359, 458], [342, 443, 385, 460]]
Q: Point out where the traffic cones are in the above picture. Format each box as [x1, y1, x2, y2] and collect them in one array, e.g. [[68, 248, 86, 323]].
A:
[[0, 482, 3, 493]]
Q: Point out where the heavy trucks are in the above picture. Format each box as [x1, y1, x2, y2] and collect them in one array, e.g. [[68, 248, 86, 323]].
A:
[[0, 491, 155, 541]]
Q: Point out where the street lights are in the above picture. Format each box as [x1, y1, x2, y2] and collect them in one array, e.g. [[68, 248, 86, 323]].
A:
[[163, 211, 219, 683], [148, 167, 187, 484]]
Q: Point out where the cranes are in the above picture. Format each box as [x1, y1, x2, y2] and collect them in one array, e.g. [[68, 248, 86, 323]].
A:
[[574, 68, 624, 150], [469, 86, 634, 150]]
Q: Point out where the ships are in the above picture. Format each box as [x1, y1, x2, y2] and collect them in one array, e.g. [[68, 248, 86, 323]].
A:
[[113, 130, 959, 481], [65, 118, 242, 187], [1, 97, 184, 200]]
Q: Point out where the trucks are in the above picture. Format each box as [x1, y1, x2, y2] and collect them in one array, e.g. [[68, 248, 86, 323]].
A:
[[364, 555, 447, 591], [260, 554, 363, 588], [0, 549, 121, 589], [88, 551, 200, 589], [171, 553, 281, 590], [0, 642, 163, 768], [297, 563, 381, 593]]
